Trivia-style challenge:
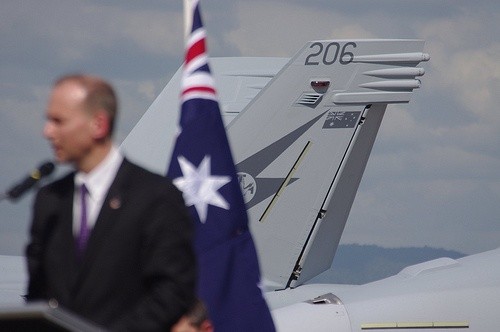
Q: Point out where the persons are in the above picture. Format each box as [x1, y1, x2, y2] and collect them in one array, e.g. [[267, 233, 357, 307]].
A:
[[24, 76, 199, 332], [170, 297, 215, 332]]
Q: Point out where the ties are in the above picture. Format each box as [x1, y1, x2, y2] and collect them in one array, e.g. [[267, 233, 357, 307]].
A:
[[77, 185, 90, 257]]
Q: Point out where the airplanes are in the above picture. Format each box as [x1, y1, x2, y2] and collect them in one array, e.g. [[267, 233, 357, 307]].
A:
[[1, 39, 499, 330]]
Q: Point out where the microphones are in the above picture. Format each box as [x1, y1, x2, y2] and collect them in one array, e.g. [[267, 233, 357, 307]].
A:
[[8, 162, 54, 198]]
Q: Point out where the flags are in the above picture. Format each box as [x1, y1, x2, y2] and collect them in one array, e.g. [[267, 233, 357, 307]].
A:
[[164, 0, 275, 332]]
